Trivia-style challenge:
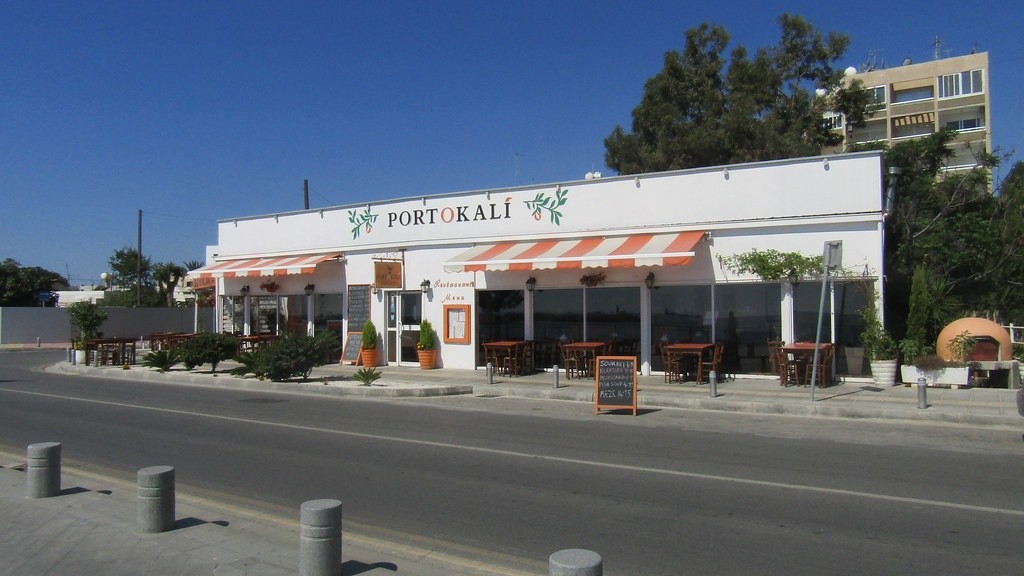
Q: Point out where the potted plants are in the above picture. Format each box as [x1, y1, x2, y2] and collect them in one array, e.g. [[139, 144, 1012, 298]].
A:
[[580, 272, 607, 287], [417, 320, 437, 369], [259, 280, 279, 294], [857, 308, 898, 386], [361, 319, 378, 367], [898, 330, 978, 385]]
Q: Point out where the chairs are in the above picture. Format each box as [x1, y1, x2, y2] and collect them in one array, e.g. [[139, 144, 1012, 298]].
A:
[[588, 336, 639, 380], [560, 339, 588, 380], [503, 338, 556, 377], [482, 340, 506, 376], [226, 331, 279, 355], [84, 336, 137, 366], [658, 341, 696, 384], [699, 340, 726, 383], [775, 341, 826, 388], [151, 333, 197, 350]]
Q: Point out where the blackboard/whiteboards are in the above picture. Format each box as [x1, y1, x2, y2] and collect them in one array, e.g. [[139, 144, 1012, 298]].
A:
[[347, 284, 371, 336], [341, 331, 364, 363], [594, 356, 637, 409]]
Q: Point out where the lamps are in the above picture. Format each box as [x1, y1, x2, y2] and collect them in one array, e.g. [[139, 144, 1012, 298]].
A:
[[787, 268, 799, 286], [370, 283, 379, 295], [305, 283, 314, 296], [240, 285, 249, 297], [645, 271, 655, 289], [420, 279, 430, 294], [526, 275, 536, 292]]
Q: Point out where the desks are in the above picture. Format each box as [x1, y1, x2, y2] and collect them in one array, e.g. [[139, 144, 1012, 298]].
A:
[[664, 343, 715, 384], [767, 340, 782, 375], [92, 338, 134, 364], [155, 333, 175, 338], [779, 342, 832, 385], [174, 334, 194, 338], [482, 341, 526, 378], [564, 341, 605, 379], [235, 335, 276, 354]]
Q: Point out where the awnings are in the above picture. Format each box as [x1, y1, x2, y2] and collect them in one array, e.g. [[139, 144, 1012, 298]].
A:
[[444, 231, 706, 272], [187, 253, 343, 278]]
